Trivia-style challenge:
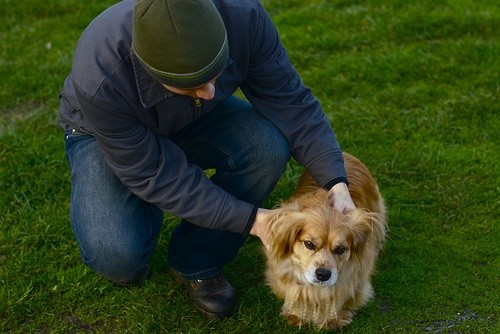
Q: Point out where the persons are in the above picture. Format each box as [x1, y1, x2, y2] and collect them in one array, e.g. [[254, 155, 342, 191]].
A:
[[57, 0, 360, 318]]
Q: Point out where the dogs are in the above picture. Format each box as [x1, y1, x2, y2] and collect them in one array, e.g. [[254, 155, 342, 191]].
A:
[[259, 150, 389, 332]]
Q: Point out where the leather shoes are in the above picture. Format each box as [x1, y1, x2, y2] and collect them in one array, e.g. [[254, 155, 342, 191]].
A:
[[115, 263, 152, 287], [164, 266, 236, 317]]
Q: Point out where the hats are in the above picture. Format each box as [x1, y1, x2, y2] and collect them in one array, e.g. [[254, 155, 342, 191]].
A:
[[133, 0, 230, 87]]
